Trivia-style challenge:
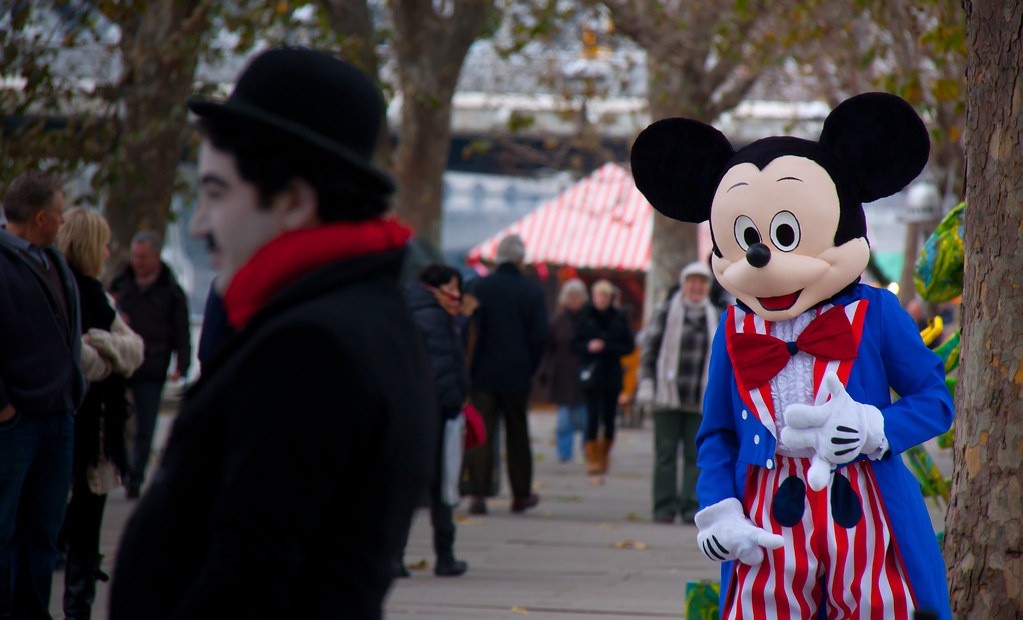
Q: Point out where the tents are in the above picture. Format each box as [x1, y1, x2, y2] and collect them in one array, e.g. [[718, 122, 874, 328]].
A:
[[467, 161, 715, 413]]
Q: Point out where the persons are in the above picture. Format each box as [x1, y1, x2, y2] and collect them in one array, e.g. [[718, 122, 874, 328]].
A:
[[0, 44, 728, 620]]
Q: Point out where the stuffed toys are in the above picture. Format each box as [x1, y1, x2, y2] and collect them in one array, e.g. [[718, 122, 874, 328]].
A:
[[630, 90, 957, 620]]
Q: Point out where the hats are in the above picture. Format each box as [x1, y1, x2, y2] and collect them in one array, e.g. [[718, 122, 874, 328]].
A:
[[187, 45, 388, 194], [680, 261, 712, 284]]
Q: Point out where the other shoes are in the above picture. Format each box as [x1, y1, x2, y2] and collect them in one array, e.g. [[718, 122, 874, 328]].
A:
[[125, 468, 143, 499], [511, 493, 539, 513], [436, 560, 467, 576], [398, 567, 409, 577], [470, 503, 485, 515]]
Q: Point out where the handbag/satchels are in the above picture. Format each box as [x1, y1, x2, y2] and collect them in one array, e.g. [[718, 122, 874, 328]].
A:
[[461, 403, 484, 449]]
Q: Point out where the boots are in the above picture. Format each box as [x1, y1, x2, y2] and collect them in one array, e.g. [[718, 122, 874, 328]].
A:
[[601, 440, 613, 472], [586, 440, 603, 472]]
